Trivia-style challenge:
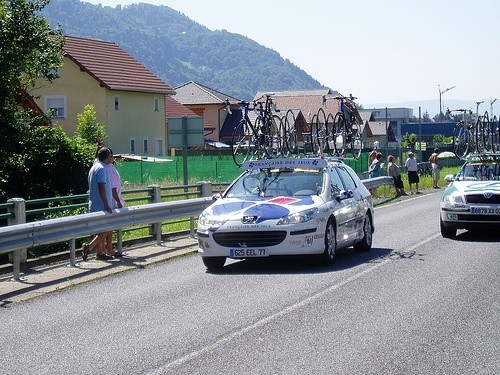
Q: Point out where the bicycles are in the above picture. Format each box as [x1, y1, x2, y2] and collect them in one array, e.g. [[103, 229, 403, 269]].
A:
[[309, 93, 366, 159], [230, 92, 298, 167], [448, 97, 500, 159]]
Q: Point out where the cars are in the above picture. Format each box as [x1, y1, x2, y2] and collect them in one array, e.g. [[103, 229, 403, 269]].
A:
[[439, 159, 500, 238], [195, 158, 377, 271]]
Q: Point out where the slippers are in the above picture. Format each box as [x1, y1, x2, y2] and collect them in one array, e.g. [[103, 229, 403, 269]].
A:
[[111, 252, 122, 257]]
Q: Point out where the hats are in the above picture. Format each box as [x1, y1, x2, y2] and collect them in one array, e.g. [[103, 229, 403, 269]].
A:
[[434, 148, 440, 152], [408, 152, 414, 156], [388, 155, 394, 159]]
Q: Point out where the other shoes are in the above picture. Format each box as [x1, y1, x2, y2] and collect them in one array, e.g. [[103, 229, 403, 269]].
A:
[[403, 193, 408, 195], [433, 186, 440, 189], [411, 192, 413, 195], [416, 192, 421, 194]]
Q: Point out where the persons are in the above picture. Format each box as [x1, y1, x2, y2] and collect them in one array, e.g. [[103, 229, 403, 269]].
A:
[[405, 152, 422, 194], [387, 154, 409, 196], [369, 146, 378, 166], [93, 146, 125, 257], [82, 146, 114, 261], [428, 147, 440, 188], [368, 152, 382, 198]]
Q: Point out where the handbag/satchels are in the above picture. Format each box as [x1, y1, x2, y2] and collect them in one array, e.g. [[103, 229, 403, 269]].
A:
[[430, 155, 438, 162]]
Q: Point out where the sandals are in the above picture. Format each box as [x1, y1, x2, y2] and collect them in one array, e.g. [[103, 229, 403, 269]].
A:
[[81, 243, 89, 260], [95, 252, 113, 260]]
